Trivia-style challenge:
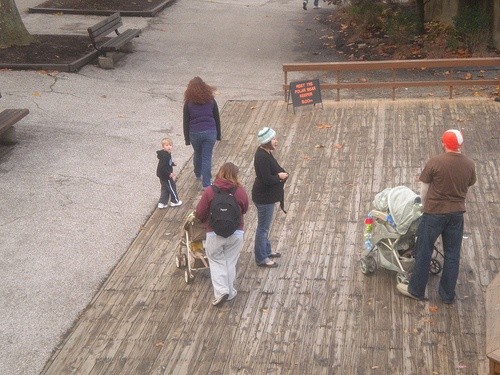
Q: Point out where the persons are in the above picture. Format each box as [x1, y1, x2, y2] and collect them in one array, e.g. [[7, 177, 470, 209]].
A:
[[196, 162, 249, 306], [183, 76, 222, 187], [302, 0, 321, 10], [396, 129, 477, 306], [156, 138, 182, 209], [252, 127, 289, 268]]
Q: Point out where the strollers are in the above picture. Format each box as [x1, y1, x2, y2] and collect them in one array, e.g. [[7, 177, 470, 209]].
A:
[[359, 185, 443, 285], [175, 209, 210, 284]]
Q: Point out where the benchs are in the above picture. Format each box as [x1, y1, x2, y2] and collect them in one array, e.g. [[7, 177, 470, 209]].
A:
[[0, 92, 29, 144], [87, 12, 142, 69]]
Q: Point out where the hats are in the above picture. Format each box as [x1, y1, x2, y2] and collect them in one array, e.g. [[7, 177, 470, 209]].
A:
[[257, 127, 276, 145], [442, 129, 463, 151]]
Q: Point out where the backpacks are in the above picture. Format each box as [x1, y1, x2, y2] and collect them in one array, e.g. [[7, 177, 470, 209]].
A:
[[208, 185, 241, 238]]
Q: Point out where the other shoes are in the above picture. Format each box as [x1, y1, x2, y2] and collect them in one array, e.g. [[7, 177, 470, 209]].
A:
[[158, 203, 168, 209], [170, 200, 183, 206], [212, 294, 229, 307], [269, 252, 281, 258], [396, 283, 422, 301], [258, 260, 278, 267]]
